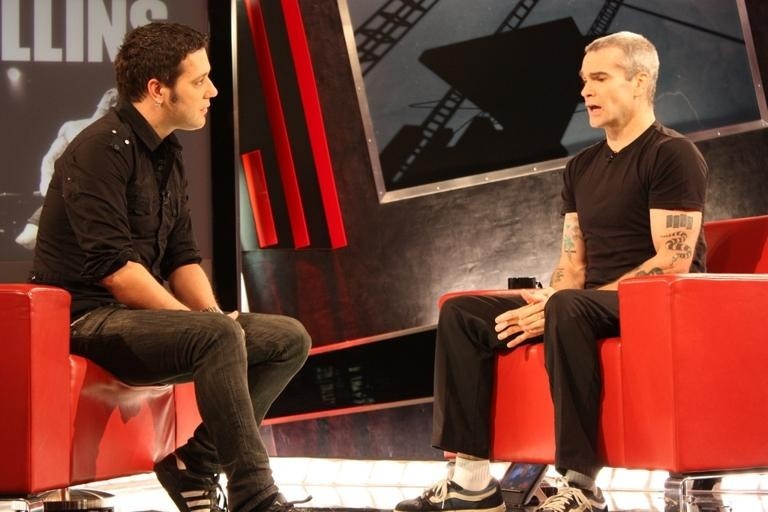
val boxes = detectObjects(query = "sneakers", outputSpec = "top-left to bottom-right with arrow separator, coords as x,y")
393,477 -> 508,511
537,481 -> 609,512
153,452 -> 227,511
251,492 -> 300,512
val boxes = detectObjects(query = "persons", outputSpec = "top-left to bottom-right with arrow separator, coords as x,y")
27,19 -> 319,511
384,25 -> 713,512
11,83 -> 123,252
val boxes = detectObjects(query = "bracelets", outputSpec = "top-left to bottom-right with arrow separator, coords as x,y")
197,305 -> 222,316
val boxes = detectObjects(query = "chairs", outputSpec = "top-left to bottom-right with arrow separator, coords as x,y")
0,280 -> 203,511
434,214 -> 768,512
